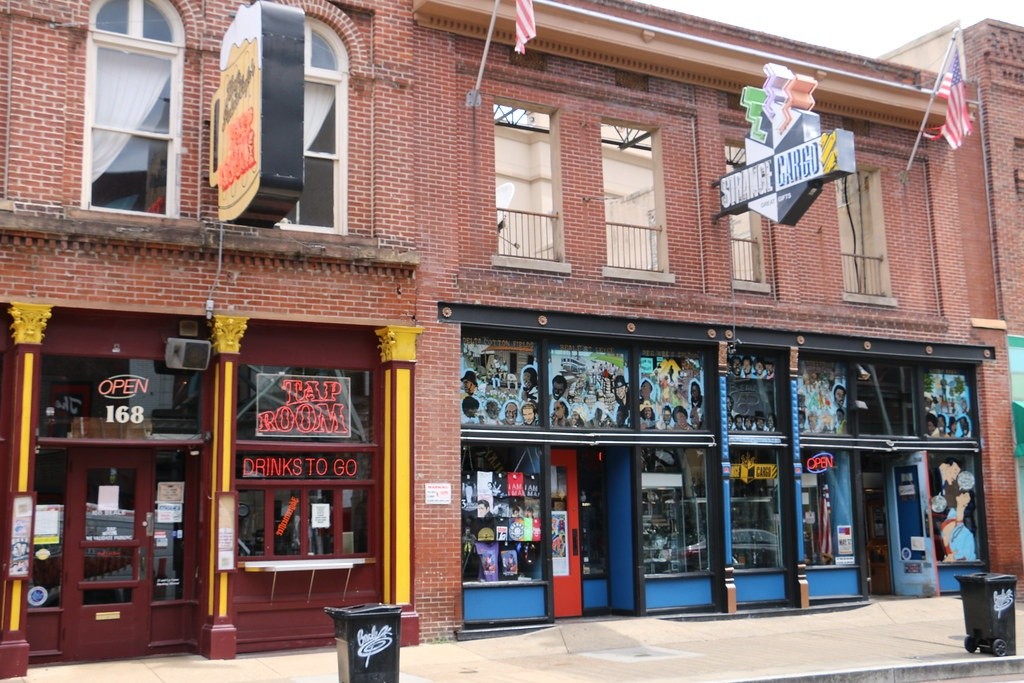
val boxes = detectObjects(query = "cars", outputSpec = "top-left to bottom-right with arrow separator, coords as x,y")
674,529 -> 782,568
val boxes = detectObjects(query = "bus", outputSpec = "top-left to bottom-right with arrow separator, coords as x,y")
676,498 -> 778,546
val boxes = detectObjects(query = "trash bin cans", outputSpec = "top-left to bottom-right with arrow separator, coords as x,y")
323,603 -> 402,683
953,573 -> 1017,657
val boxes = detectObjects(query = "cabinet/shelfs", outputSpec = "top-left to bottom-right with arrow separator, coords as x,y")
460,453 -> 822,582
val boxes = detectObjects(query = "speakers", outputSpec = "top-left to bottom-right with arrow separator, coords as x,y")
165,338 -> 212,370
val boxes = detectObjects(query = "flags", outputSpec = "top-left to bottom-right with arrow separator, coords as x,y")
513,0 -> 536,55
921,40 -> 971,149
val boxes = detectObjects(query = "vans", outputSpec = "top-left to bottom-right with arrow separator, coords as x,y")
34,503 -> 175,599
